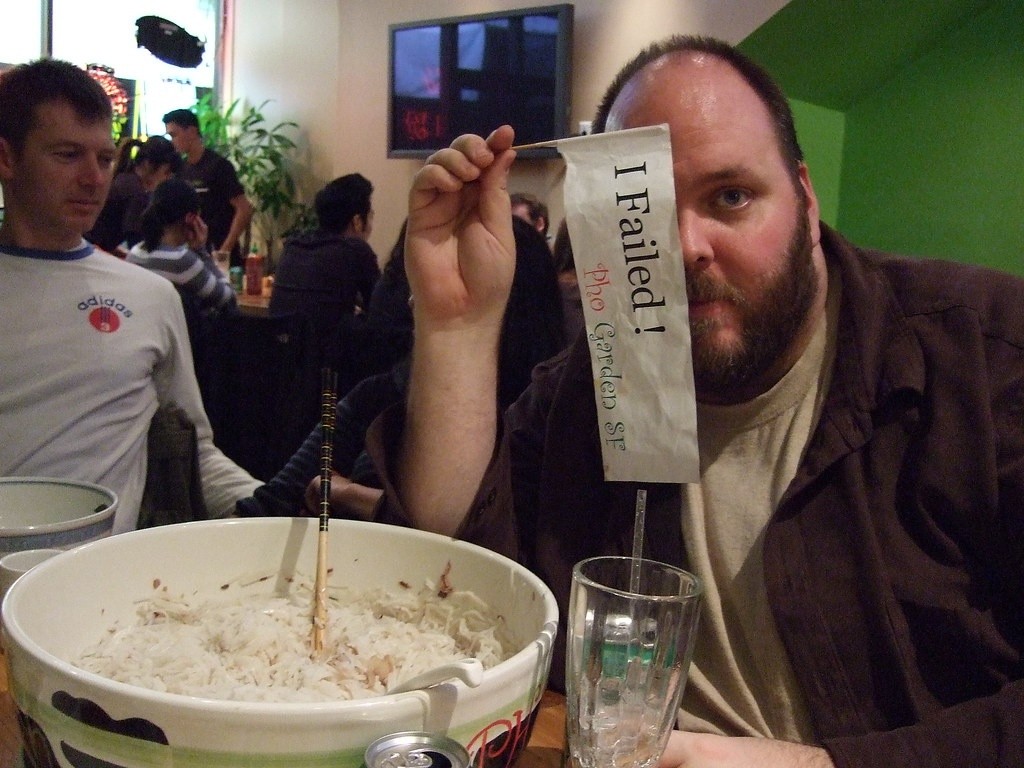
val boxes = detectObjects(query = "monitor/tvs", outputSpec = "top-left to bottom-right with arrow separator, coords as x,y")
386,3 -> 574,159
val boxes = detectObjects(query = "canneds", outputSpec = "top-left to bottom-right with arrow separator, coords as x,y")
229,266 -> 243,295
562,611 -> 663,768
359,730 -> 473,768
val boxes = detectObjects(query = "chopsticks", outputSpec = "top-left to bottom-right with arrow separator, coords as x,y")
311,365 -> 339,653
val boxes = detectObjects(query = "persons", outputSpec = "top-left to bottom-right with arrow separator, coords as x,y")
229,217 -> 567,526
83,135 -> 182,260
124,180 -> 238,317
267,173 -> 382,381
509,193 -> 550,239
365,33 -> 1024,768
162,109 -> 252,268
0,58 -> 260,534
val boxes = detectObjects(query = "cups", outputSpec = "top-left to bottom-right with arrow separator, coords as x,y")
211,250 -> 230,274
561,556 -> 705,768
0,548 -> 65,605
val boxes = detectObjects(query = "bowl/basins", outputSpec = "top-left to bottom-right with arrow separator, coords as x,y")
0,476 -> 118,560
1,516 -> 559,768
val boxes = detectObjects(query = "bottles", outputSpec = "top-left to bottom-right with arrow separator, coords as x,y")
245,241 -> 265,297
